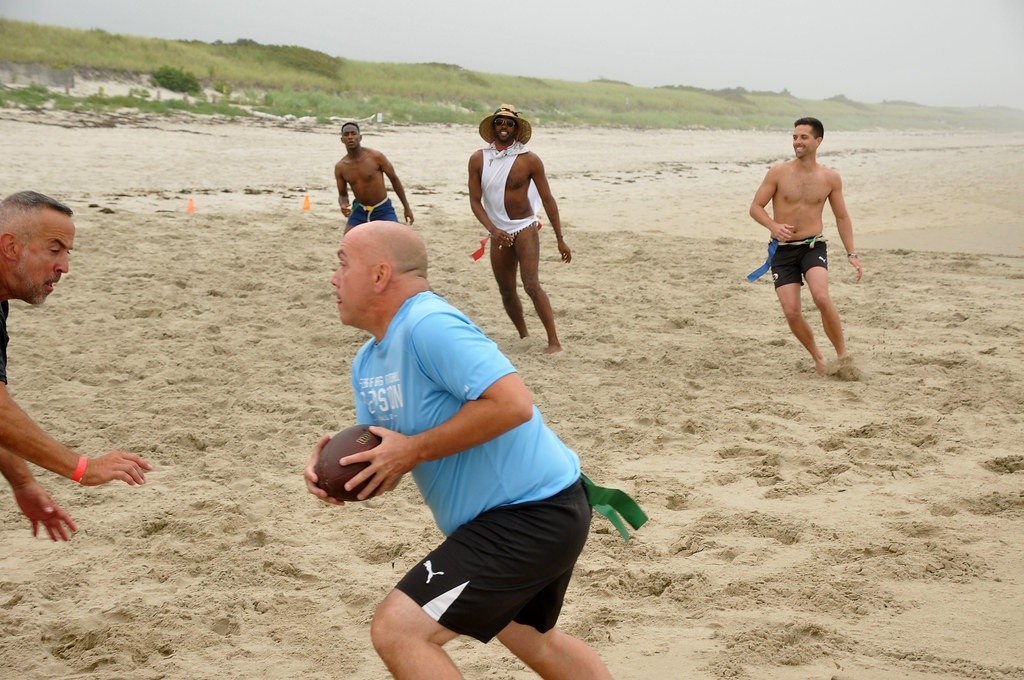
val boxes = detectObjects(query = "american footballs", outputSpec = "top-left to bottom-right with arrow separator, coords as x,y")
314,423 -> 383,505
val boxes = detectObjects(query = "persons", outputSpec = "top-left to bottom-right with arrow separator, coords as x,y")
305,218 -> 618,680
0,190 -> 153,541
467,104 -> 572,353
334,122 -> 414,234
749,117 -> 863,375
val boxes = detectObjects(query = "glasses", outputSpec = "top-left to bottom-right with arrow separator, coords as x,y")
494,118 -> 517,127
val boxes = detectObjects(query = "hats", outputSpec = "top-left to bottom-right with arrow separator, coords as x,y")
478,103 -> 532,145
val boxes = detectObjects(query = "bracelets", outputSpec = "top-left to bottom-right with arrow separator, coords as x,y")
72,457 -> 88,483
556,236 -> 564,239
847,253 -> 859,259
